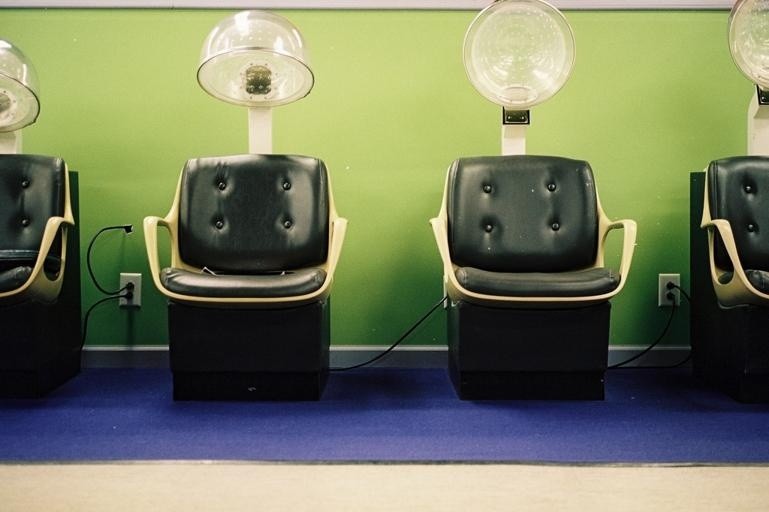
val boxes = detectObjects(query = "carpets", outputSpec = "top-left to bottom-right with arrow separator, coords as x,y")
0,367 -> 769,468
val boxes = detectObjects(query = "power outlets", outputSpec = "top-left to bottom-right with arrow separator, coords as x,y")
658,273 -> 681,307
118,272 -> 143,307
442,276 -> 448,309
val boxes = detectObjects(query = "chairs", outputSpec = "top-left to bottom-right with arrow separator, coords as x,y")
427,153 -> 637,402
142,153 -> 348,400
0,153 -> 83,399
699,154 -> 769,403
